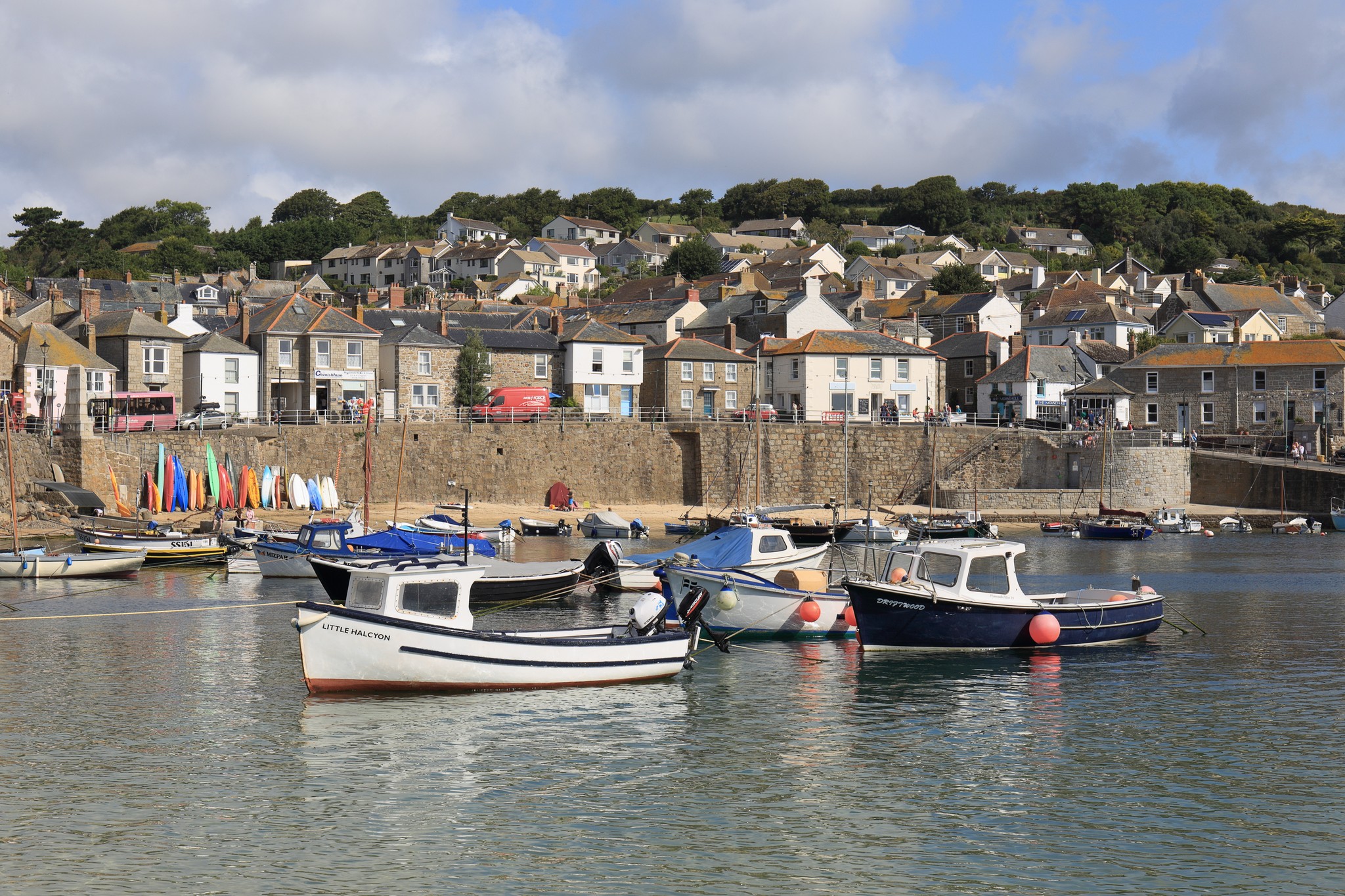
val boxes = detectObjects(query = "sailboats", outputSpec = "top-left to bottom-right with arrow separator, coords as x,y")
0,385 -> 148,579
1070,398 -> 1154,540
1272,470 -> 1291,534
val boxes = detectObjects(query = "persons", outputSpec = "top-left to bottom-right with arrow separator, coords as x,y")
1162,429 -> 1175,446
246,506 -> 258,522
1128,420 -> 1133,430
341,399 -> 347,424
271,411 -> 282,426
685,511 -> 690,526
1088,411 -> 1104,430
792,401 -> 804,425
147,403 -> 165,411
1078,431 -> 1098,447
234,507 -> 244,528
879,402 -> 899,427
213,507 -> 224,530
1290,439 -> 1304,467
8,405 -> 24,432
1188,429 -> 1198,453
1115,418 -> 1121,431
318,392 -> 327,415
923,404 -> 963,427
913,408 -> 921,422
558,488 -> 573,511
91,509 -> 103,517
1239,516 -> 1244,528
1011,409 -> 1017,428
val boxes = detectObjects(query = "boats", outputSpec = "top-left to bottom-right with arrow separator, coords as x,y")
1330,497 -> 1345,531
293,564 -> 702,694
1219,512 -> 1252,534
69,524 -> 229,568
228,398 -> 998,608
1284,524 -> 1302,535
1152,507 -> 1201,533
1289,517 -> 1323,533
1040,489 -> 1078,537
659,553 -> 857,644
834,536 -> 1168,656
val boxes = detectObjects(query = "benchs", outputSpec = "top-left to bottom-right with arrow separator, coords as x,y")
1225,436 -> 1256,448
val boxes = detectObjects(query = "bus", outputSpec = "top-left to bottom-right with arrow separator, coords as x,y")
86,391 -> 176,433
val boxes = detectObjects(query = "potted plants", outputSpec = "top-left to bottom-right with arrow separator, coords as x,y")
1237,429 -> 1249,435
1014,332 -> 1022,335
1273,417 -> 1284,436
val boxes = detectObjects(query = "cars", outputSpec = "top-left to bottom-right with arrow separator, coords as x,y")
170,402 -> 233,431
1333,445 -> 1345,465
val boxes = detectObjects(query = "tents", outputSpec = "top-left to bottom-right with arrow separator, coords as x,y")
548,393 -> 562,407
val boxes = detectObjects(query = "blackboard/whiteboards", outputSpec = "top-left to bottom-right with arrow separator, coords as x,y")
884,398 -> 895,412
858,398 -> 869,415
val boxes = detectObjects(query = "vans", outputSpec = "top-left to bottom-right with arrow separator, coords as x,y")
468,387 -> 550,423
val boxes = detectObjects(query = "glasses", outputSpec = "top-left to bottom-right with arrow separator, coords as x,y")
247,509 -> 249,510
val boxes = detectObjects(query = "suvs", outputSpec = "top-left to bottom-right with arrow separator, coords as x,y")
730,403 -> 778,423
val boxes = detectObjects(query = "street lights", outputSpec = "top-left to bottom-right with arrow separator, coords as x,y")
1128,397 -> 1134,420
1071,348 -> 1080,431
39,339 -> 51,435
